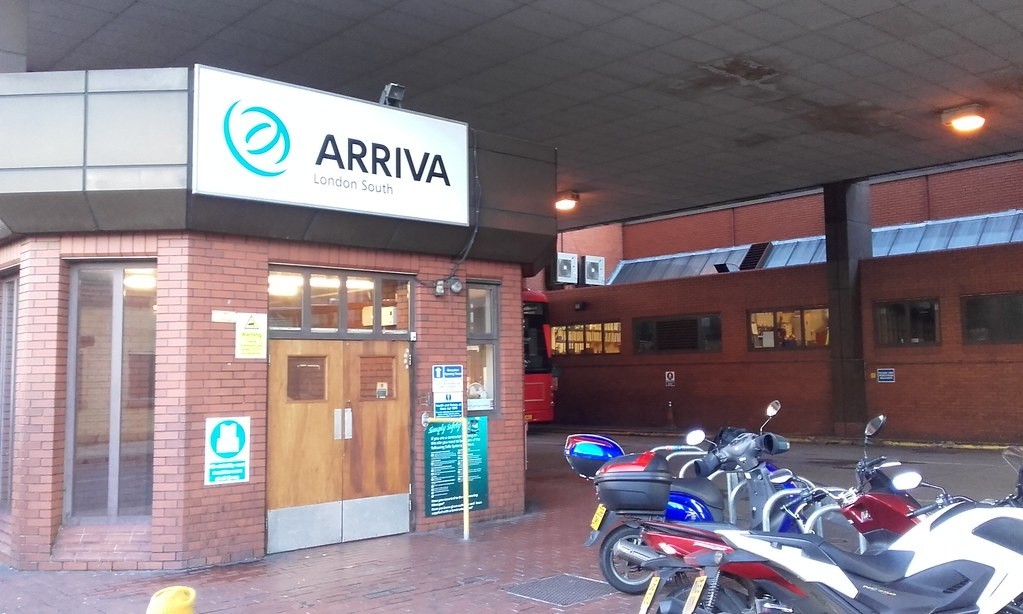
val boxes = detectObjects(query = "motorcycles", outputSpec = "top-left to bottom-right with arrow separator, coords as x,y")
636,446 -> 1023,614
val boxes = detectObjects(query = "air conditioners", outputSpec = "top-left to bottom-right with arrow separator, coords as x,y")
545,249 -> 579,284
575,256 -> 606,288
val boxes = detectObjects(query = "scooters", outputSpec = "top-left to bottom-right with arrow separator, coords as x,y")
564,398 -> 931,596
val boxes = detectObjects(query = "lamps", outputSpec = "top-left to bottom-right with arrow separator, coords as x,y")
381,82 -> 405,108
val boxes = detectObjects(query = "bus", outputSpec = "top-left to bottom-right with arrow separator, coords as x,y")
522,286 -> 558,433
